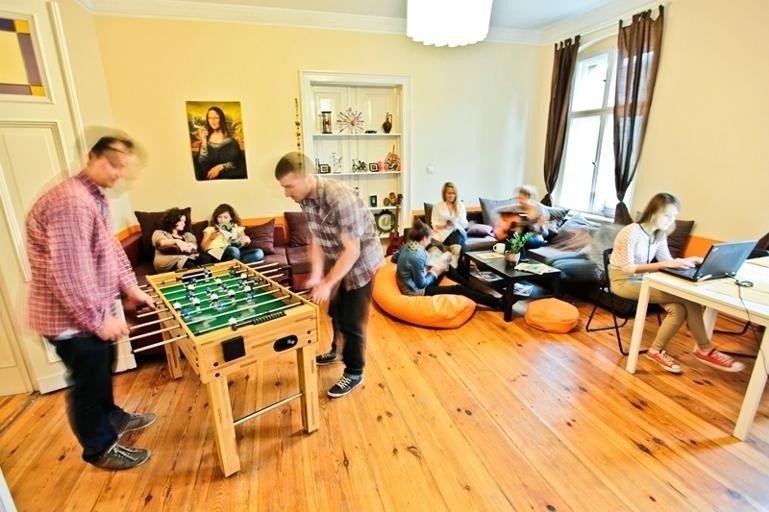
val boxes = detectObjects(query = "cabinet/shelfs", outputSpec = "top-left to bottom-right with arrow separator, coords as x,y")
294,67 -> 412,244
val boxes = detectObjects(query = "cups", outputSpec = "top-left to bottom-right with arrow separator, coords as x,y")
491,242 -> 506,254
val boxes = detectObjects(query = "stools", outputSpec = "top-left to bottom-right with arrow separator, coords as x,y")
523,297 -> 581,334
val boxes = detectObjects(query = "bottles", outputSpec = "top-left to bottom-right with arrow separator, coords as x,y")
383,113 -> 392,133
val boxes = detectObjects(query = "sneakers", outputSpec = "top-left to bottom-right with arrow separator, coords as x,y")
316,352 -> 345,366
694,347 -> 746,372
328,376 -> 364,398
117,412 -> 158,440
92,445 -> 150,470
645,349 -> 682,374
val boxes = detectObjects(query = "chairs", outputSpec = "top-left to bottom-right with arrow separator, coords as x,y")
586,245 -> 670,360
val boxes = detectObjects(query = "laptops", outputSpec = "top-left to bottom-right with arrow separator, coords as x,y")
658,240 -> 757,281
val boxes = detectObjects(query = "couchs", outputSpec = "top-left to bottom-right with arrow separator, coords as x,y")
111,217 -> 317,361
409,202 -> 621,304
371,252 -> 478,330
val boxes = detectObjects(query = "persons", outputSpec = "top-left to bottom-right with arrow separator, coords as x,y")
607,193 -> 746,372
391,182 -> 624,312
276,153 -> 387,398
199,205 -> 265,263
152,209 -> 212,273
198,107 -> 244,180
26,136 -> 157,470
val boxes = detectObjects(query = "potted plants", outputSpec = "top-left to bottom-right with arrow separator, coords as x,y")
503,230 -> 534,267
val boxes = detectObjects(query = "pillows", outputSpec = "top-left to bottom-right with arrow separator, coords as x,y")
552,212 -> 597,247
476,195 -> 519,226
634,212 -> 694,259
284,209 -> 313,249
133,207 -> 195,265
546,205 -> 571,233
423,201 -> 435,226
466,222 -> 493,238
245,216 -> 278,257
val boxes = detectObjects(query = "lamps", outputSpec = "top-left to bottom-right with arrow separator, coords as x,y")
401,1 -> 496,47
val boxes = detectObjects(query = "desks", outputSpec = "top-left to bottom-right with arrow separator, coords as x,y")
620,258 -> 768,443
